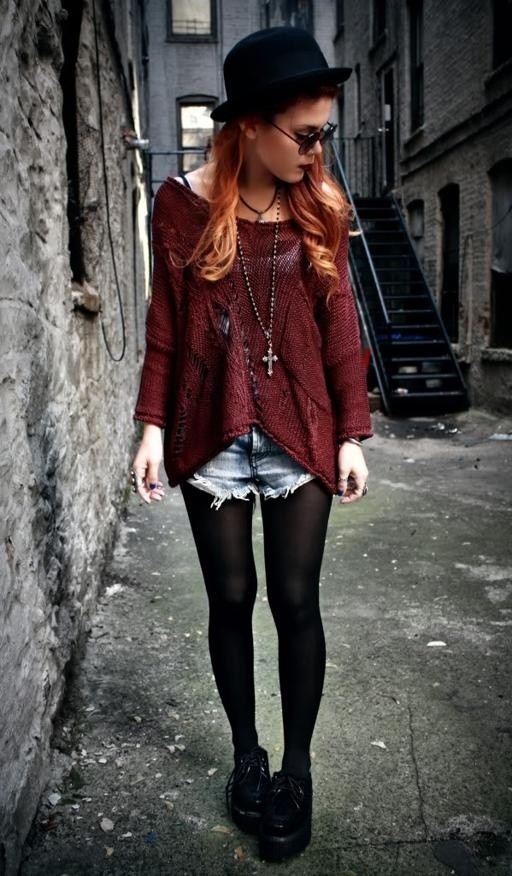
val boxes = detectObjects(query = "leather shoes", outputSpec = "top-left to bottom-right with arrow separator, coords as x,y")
225,746 -> 272,837
257,769 -> 312,864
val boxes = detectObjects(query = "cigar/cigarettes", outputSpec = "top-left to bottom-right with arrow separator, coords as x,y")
139,489 -> 151,507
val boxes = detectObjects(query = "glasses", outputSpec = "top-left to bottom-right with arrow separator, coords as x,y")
263,117 -> 339,156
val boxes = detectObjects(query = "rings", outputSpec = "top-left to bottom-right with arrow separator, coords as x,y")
339,478 -> 348,481
361,484 -> 369,495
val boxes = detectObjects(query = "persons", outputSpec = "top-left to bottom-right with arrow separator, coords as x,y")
132,28 -> 374,862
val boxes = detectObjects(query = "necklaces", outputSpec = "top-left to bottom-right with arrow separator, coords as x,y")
236,188 -> 281,378
235,182 -> 277,223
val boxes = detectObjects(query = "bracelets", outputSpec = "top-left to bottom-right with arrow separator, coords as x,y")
348,437 -> 364,446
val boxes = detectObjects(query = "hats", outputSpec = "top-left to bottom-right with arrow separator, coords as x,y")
210,24 -> 353,123
